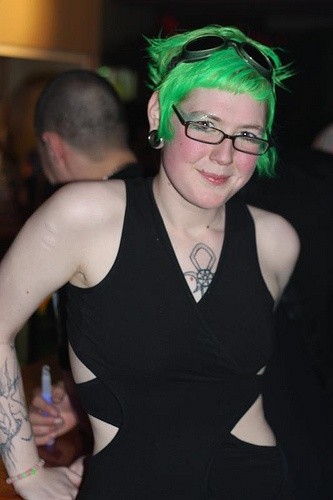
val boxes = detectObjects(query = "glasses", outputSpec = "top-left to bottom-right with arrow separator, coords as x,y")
167,35 -> 276,85
171,106 -> 273,159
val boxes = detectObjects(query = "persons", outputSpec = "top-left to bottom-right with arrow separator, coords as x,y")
35,68 -> 146,372
252,60 -> 333,500
0,27 -> 299,500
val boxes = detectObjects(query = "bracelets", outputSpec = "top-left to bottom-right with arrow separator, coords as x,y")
6,459 -> 45,483
